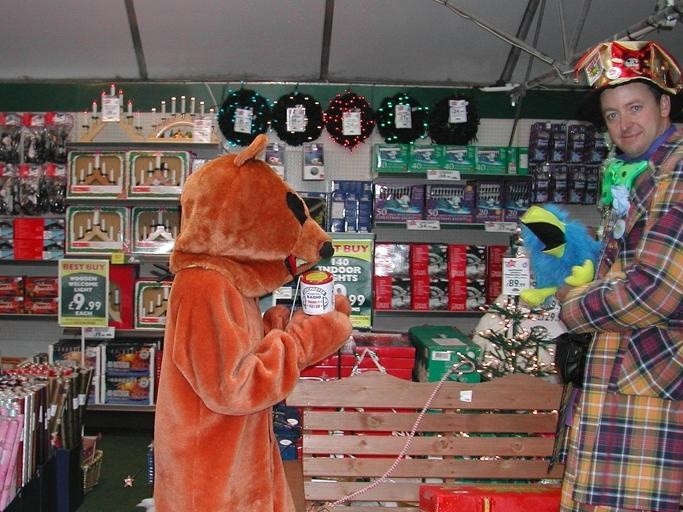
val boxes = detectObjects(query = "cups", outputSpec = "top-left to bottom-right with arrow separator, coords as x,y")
298,270 -> 347,316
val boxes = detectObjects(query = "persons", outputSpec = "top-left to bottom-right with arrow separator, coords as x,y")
546,42 -> 683,511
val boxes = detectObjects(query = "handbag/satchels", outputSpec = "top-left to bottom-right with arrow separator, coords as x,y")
554,332 -> 592,389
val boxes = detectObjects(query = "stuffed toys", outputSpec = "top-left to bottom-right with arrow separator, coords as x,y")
516,200 -> 605,307
152,137 -> 358,511
